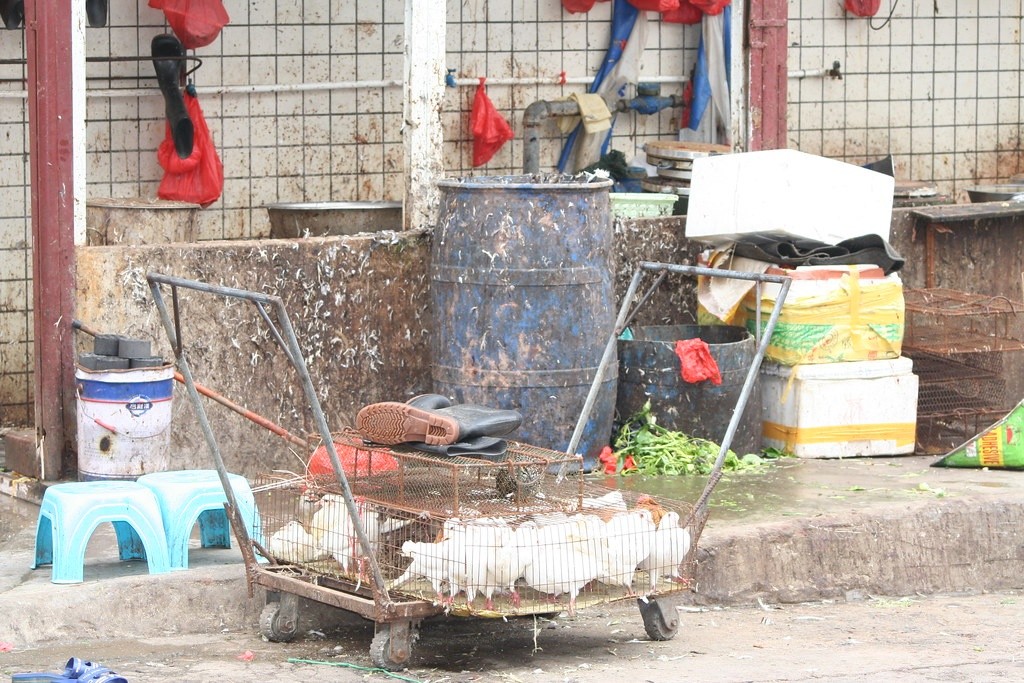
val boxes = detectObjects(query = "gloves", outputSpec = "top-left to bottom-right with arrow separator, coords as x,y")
557,93 -> 612,134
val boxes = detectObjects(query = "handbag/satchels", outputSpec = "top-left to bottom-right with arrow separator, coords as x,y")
310,436 -> 399,485
148,0 -> 230,49
158,90 -> 223,208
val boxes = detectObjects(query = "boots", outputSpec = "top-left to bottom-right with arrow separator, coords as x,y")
356,402 -> 522,444
363,391 -> 508,457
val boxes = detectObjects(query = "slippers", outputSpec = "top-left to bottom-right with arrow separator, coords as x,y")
12,657 -> 129,683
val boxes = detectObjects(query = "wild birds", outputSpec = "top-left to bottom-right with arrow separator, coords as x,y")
263,488 -> 433,593
378,487 -> 692,610
494,465 -> 536,505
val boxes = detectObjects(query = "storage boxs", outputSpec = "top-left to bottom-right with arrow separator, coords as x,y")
759,355 -> 919,459
685,149 -> 895,249
698,250 -> 905,364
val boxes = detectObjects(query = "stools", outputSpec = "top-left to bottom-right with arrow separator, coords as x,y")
137,470 -> 267,570
30,481 -> 172,584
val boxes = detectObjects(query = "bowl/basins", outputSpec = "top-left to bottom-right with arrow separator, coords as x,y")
262,199 -> 404,237
609,190 -> 679,220
963,182 -> 1024,204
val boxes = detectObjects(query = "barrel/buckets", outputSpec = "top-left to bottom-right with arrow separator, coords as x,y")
614,326 -> 765,463
66,361 -> 175,485
88,197 -> 200,248
426,173 -> 620,475
